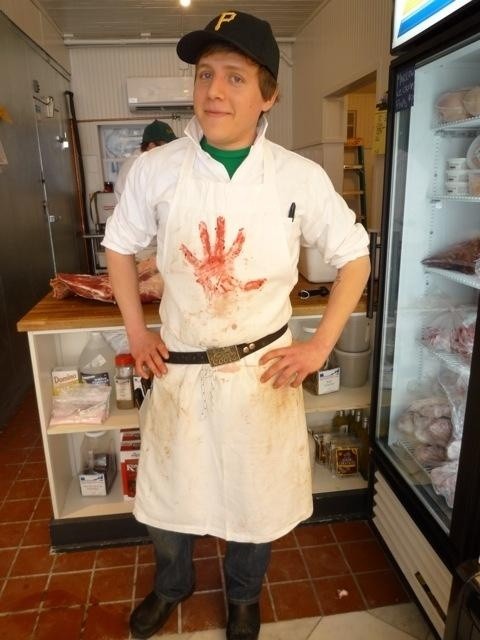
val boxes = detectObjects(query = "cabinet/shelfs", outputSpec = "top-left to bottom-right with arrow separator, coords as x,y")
395,110 -> 479,520
17,287 -> 377,554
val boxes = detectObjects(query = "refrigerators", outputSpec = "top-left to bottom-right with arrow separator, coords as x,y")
368,0 -> 480,640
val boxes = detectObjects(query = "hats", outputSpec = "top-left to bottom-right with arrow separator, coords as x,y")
142,119 -> 179,143
176,10 -> 279,81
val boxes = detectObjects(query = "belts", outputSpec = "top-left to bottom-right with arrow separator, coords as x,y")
163,321 -> 289,368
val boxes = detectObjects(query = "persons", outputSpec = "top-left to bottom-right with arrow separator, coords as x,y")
100,11 -> 371,640
113,119 -> 179,200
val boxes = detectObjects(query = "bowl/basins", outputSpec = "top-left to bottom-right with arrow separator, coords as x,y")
446,181 -> 467,195
446,170 -> 467,181
447,157 -> 467,169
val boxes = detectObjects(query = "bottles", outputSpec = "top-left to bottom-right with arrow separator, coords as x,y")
332,408 -> 371,483
110,353 -> 135,408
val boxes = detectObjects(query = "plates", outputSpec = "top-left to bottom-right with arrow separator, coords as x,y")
467,135 -> 480,170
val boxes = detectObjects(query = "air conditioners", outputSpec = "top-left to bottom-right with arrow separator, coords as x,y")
124,75 -> 195,116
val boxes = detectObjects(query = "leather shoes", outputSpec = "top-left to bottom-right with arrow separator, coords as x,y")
130,582 -> 196,639
225,602 -> 261,640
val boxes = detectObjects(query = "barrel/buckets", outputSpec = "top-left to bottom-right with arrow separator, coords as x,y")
80,331 -> 117,403
296,322 -> 340,395
77,431 -> 118,496
